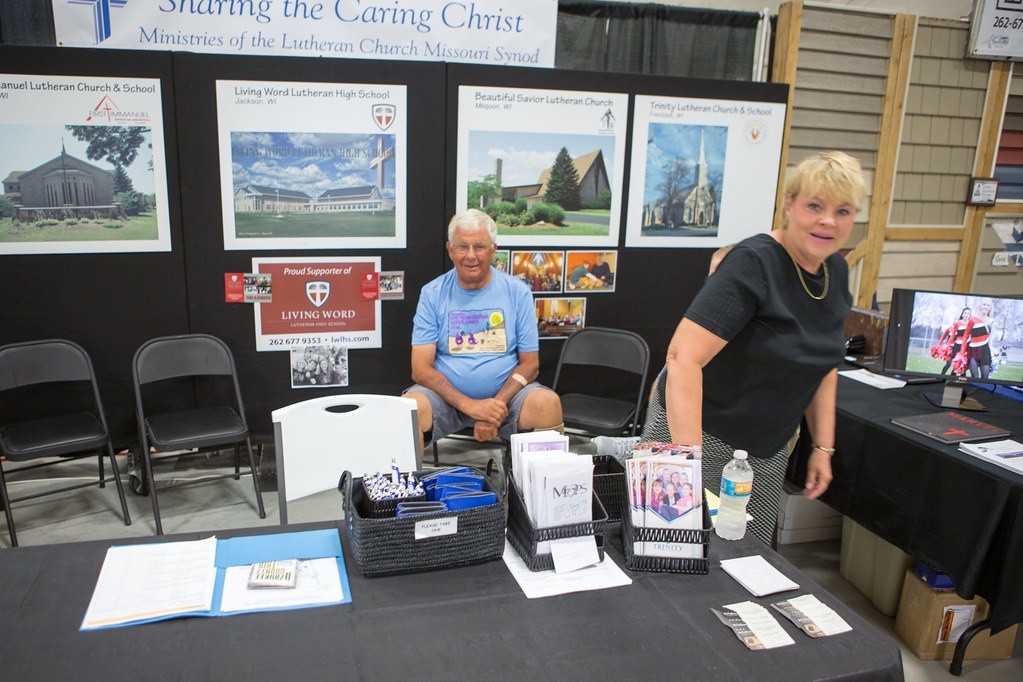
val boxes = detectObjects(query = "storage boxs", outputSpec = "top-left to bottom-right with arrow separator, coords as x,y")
777,477 -> 1018,661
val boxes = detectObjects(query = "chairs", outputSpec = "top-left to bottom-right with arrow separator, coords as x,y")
131,334 -> 265,536
0,337 -> 130,548
271,394 -> 423,526
421,327 -> 650,473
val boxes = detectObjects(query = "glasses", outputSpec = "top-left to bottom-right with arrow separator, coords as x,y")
450,240 -> 495,254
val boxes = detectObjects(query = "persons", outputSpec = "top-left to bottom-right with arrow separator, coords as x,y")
937,299 -> 995,380
401,209 -> 565,463
293,355 -> 347,385
631,462 -> 693,521
590,435 -> 641,466
568,257 -> 610,290
538,314 -> 579,337
492,259 -> 507,274
641,150 -> 869,546
520,272 -> 560,291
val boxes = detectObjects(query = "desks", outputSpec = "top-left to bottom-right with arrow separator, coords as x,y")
0,520 -> 907,682
787,353 -> 1023,677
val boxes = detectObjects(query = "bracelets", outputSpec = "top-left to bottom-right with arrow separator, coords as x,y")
813,444 -> 835,456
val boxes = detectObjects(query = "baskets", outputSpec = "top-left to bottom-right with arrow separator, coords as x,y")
507,455 -> 714,575
338,456 -> 507,578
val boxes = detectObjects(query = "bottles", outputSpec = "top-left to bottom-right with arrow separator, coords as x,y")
715,450 -> 753,541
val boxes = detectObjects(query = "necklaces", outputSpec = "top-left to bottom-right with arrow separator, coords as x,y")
782,229 -> 830,299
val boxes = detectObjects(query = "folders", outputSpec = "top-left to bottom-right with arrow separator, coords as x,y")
80,527 -> 352,633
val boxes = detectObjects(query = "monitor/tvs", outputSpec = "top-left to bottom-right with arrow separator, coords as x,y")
882,288 -> 1023,411
